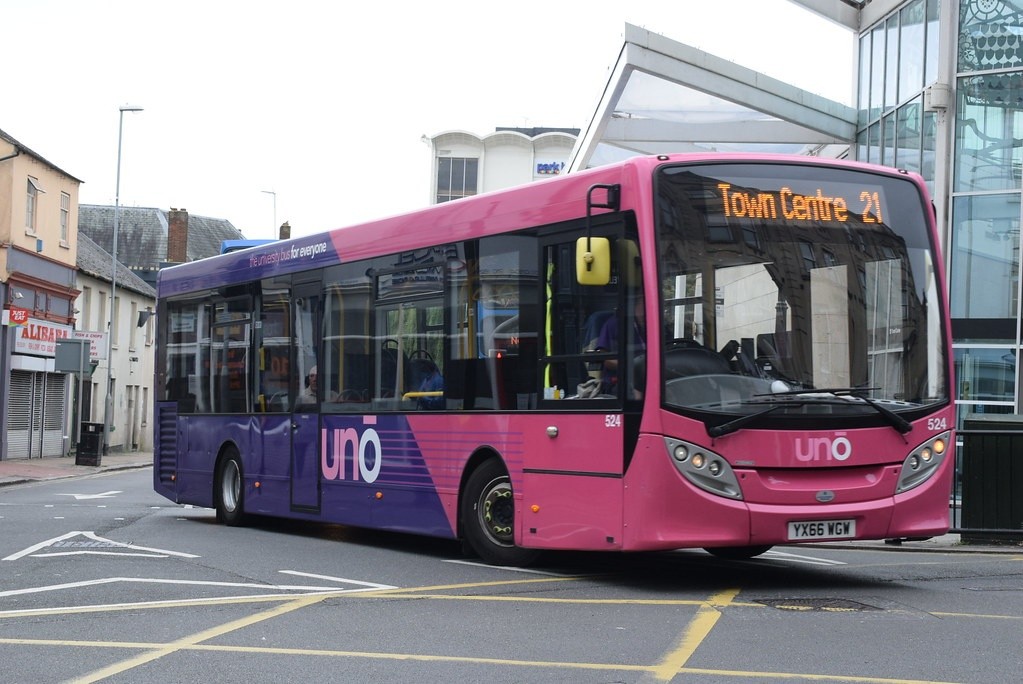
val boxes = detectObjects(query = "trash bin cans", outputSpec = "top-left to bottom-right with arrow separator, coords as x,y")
961,414 -> 1023,544
75,421 -> 105,466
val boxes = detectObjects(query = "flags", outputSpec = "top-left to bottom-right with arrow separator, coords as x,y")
137,312 -> 151,328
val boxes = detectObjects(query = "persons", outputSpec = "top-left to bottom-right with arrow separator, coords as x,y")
295,364 -> 343,404
594,285 -> 665,397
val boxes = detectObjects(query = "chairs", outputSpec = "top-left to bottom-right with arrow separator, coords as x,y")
166,309 -> 617,413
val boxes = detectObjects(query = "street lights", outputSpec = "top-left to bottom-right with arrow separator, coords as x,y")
104,103 -> 143,453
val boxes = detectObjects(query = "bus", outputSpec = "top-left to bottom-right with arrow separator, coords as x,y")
153,151 -> 961,564
